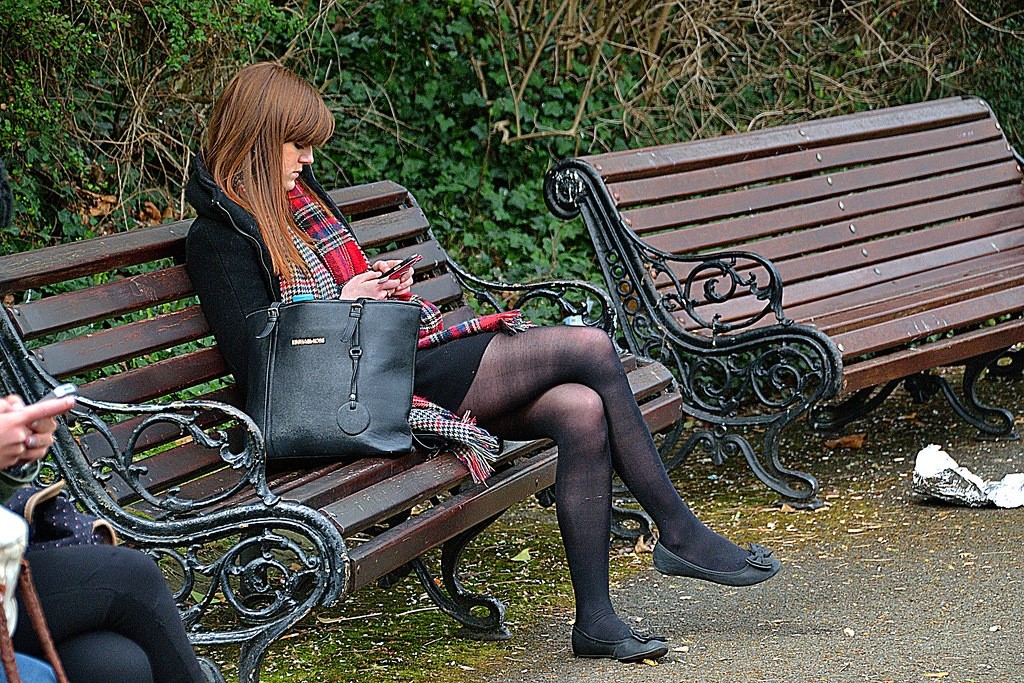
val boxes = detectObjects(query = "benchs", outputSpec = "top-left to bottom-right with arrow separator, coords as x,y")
0,179 -> 687,683
542,94 -> 1024,500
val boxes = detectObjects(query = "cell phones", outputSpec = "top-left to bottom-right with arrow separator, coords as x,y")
376,253 -> 417,278
36,382 -> 80,405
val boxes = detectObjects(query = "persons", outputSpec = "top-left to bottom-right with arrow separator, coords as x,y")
0,395 -> 206,683
185,62 -> 781,660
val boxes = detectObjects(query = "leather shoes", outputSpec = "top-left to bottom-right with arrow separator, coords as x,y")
568,622 -> 668,662
652,537 -> 783,588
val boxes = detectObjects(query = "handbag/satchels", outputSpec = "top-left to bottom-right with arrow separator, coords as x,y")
0,508 -> 31,647
5,478 -> 117,546
246,300 -> 422,461
